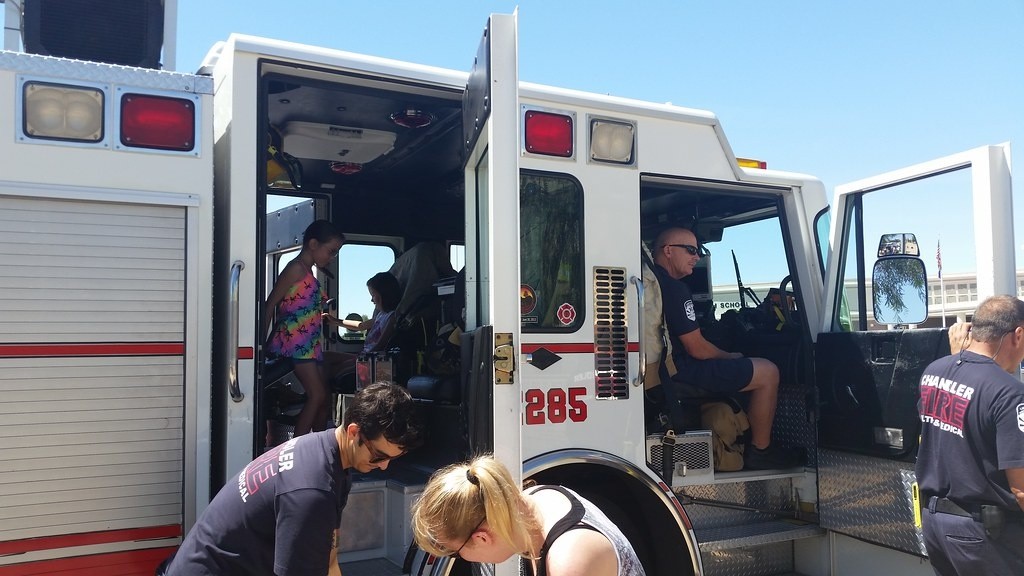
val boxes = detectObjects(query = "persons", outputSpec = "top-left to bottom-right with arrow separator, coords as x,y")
915,295 -> 1024,576
322,271 -> 401,395
651,227 -> 800,471
265,219 -> 344,437
165,380 -> 424,576
411,453 -> 645,576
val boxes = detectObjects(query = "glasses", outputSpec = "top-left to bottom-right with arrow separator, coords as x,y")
448,516 -> 486,559
323,245 -> 339,258
662,245 -> 702,257
358,431 -> 408,464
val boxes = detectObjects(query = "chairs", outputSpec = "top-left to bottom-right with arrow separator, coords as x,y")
334,242 -> 465,405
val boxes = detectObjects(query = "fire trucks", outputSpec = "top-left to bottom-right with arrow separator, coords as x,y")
0,4 -> 1024,576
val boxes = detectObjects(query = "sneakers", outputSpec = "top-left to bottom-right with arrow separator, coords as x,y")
746,442 -> 785,470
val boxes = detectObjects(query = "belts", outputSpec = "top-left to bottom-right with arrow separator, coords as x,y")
924,495 -> 1024,521
